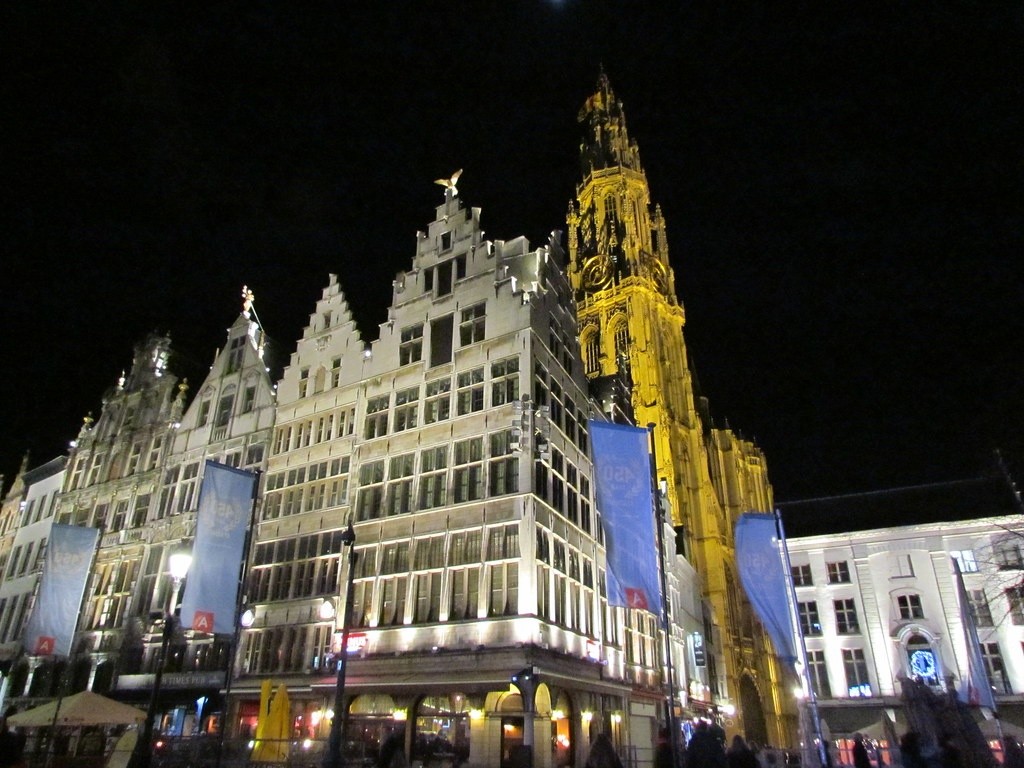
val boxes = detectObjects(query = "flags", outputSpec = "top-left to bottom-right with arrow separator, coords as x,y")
179,459 -> 257,636
22,523 -> 100,657
589,419 -> 667,628
736,513 -> 798,660
951,556 -> 999,711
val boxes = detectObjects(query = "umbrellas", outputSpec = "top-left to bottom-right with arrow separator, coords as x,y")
7,689 -> 148,760
251,680 -> 291,762
882,677 -> 1003,767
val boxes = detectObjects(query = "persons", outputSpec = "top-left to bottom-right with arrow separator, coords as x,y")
653,717 -> 762,768
851,736 -> 870,768
585,733 -> 624,768
